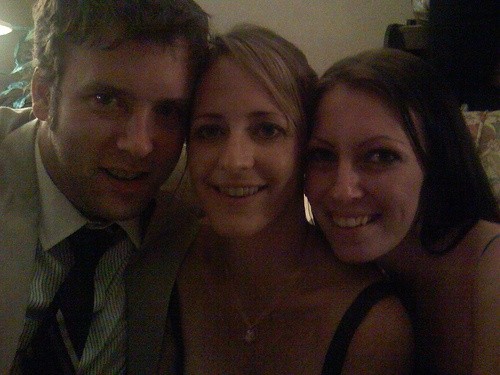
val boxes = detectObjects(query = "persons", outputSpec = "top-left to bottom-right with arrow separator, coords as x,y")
304,48 -> 500,375
157,25 -> 414,375
0,0 -> 208,375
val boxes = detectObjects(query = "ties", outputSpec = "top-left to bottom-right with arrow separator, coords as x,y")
19,224 -> 125,375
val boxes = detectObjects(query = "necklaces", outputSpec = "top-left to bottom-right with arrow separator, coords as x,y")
221,241 -> 309,345
